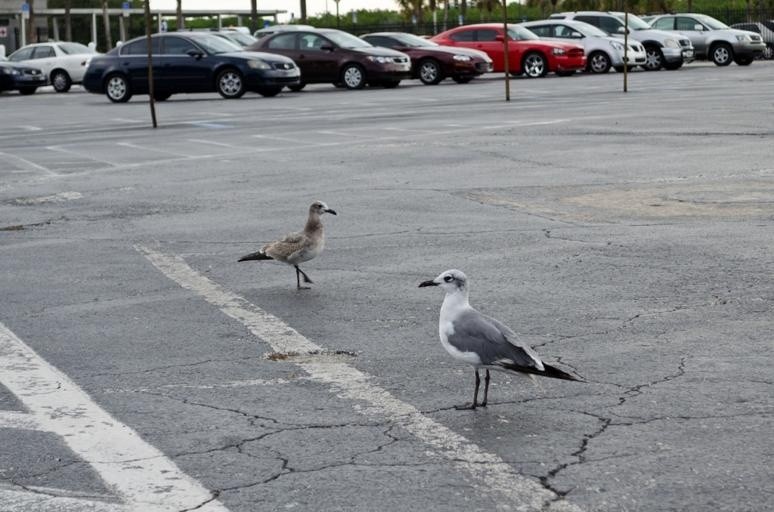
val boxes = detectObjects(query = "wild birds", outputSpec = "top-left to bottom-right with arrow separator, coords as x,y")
237,199 -> 337,290
418,268 -> 590,410
